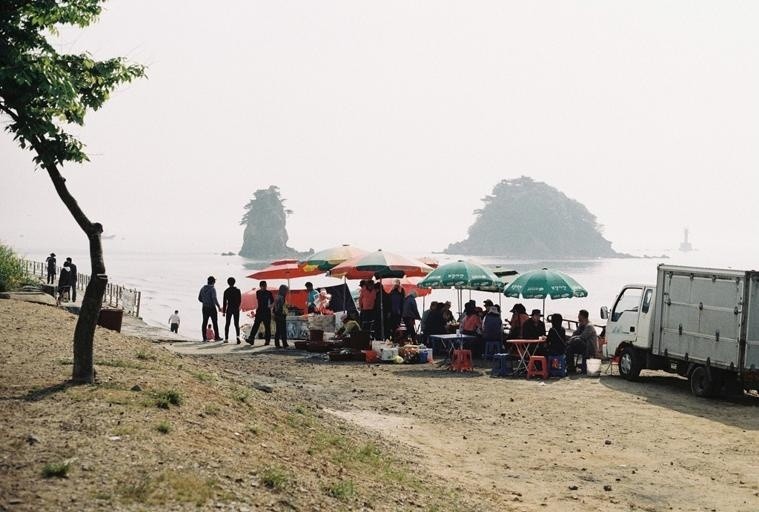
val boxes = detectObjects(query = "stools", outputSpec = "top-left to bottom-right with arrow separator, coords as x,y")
527,356 -> 549,380
490,353 -> 514,378
575,352 -> 595,375
548,354 -> 568,379
485,341 -> 500,359
453,348 -> 473,375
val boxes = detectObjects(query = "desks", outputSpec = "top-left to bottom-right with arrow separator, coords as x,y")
506,339 -> 547,377
430,334 -> 477,371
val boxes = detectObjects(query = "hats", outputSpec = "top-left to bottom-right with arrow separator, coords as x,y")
488,307 -> 499,315
528,310 -> 544,317
339,314 -> 347,322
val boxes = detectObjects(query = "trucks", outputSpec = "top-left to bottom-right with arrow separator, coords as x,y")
598,260 -> 759,406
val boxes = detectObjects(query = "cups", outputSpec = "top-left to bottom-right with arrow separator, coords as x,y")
539,336 -> 542,341
456,328 -> 460,336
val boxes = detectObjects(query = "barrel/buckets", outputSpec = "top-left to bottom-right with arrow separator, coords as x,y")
585,358 -> 601,377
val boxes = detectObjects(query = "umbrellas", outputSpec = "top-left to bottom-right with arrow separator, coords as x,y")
503,266 -> 588,322
297,244 -> 371,313
416,260 -> 503,315
219,287 -> 257,312
325,249 -> 436,337
246,258 -> 325,306
492,265 -> 520,306
419,258 -> 439,312
375,277 -> 431,297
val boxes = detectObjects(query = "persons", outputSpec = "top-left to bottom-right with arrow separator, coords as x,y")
66,257 -> 77,302
168,310 -> 180,333
329,289 -> 348,330
206,324 -> 215,340
198,276 -> 223,341
247,311 -> 256,318
223,277 -> 241,344
58,261 -> 72,298
46,253 -> 56,284
305,281 -> 320,314
358,279 -> 599,373
245,281 -> 274,346
273,284 -> 289,348
308,288 -> 333,315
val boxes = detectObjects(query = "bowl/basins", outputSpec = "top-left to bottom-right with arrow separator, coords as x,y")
327,351 -> 352,360
293,340 -> 333,352
